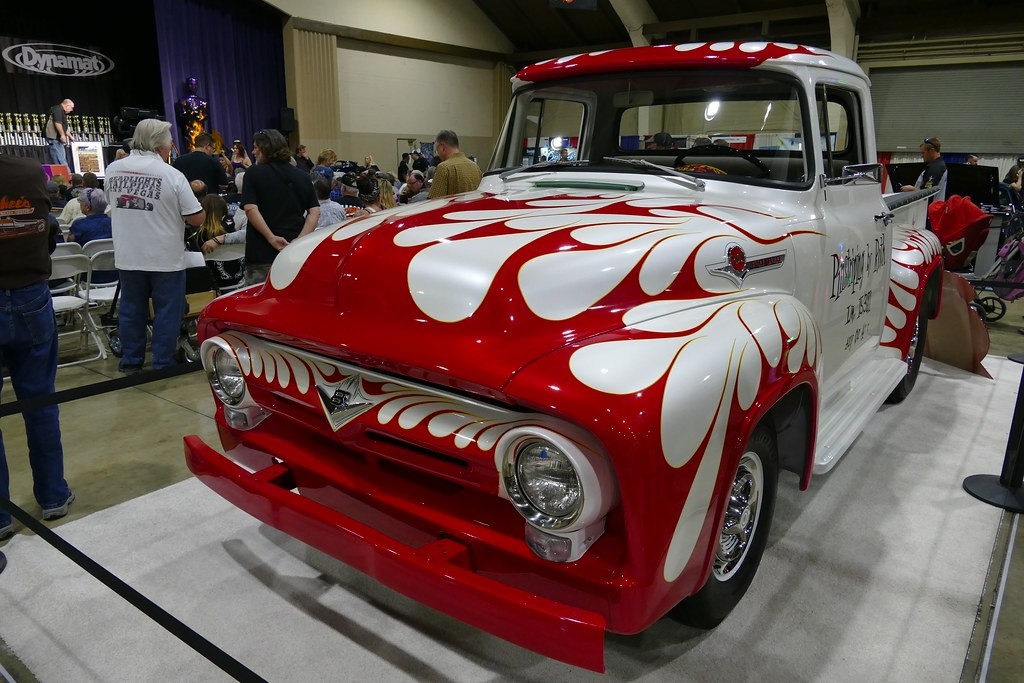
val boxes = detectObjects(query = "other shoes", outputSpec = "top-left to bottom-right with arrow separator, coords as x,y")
118,361 -> 142,373
0,521 -> 15,539
42,491 -> 75,519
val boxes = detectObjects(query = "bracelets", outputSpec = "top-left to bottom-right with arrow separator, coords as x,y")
212,237 -> 223,245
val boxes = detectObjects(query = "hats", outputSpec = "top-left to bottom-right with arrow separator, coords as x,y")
337,173 -> 357,188
48,182 -> 58,193
645,132 -> 672,146
1018,158 -> 1024,164
409,150 -> 418,155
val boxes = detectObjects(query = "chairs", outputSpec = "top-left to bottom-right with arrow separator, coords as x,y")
46,219 -> 217,367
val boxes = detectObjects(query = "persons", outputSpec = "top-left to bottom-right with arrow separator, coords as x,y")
899,138 -> 947,231
240,129 -> 321,289
540,156 -> 547,163
1001,158 -> 1024,239
173,131 -> 401,298
40,99 -> 75,174
0,153 -> 75,542
103,119 -> 206,373
114,149 -> 128,162
646,131 -> 678,149
548,154 -> 552,161
713,139 -> 729,148
176,77 -> 207,153
559,149 -> 569,161
428,130 -> 483,200
44,172 -> 118,327
397,150 -> 442,205
693,138 -> 712,149
967,154 -> 978,165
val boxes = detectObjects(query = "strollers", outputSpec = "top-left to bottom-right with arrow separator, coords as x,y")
100,226 -> 221,371
928,195 -> 1024,322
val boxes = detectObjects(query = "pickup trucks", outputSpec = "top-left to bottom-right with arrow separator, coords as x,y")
184,42 -> 946,674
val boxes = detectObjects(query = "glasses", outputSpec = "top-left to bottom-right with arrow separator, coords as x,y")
434,145 -> 444,154
76,199 -> 84,202
206,144 -> 216,153
259,128 -> 267,136
924,138 -> 938,149
409,179 -> 418,184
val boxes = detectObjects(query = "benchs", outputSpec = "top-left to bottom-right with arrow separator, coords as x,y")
613,154 -> 848,184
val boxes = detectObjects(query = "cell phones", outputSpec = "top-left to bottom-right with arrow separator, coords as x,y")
898,183 -> 903,187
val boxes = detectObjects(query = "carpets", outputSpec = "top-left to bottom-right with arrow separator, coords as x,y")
0,354 -> 1023,683
